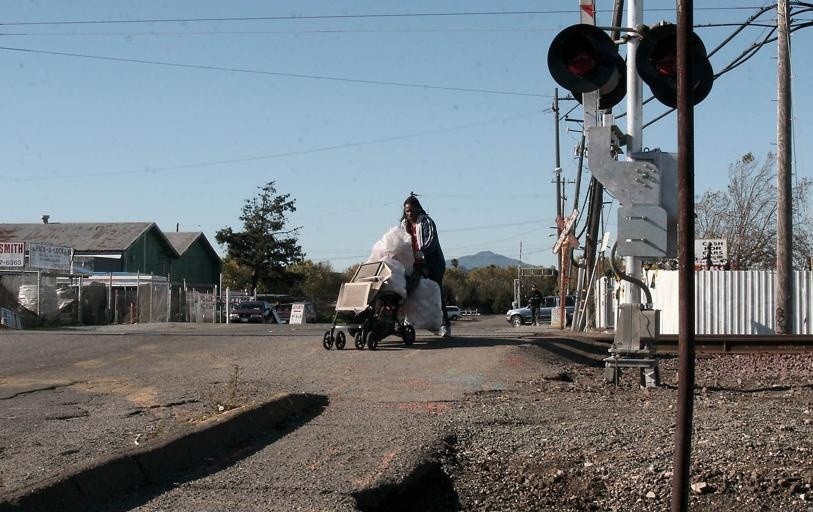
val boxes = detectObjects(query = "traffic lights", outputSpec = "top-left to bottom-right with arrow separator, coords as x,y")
549,24 -> 616,89
636,24 -> 708,91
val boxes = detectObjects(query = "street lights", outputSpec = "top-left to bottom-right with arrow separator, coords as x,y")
552,167 -> 565,288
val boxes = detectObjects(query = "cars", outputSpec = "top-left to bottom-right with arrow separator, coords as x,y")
186,292 -> 336,325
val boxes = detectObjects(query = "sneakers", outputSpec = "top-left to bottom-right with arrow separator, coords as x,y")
531,321 -> 540,326
427,324 -> 452,338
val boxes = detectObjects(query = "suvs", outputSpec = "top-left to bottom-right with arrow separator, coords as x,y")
504,294 -> 581,328
445,303 -> 462,322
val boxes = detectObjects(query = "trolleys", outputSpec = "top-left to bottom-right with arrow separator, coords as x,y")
322,252 -> 422,350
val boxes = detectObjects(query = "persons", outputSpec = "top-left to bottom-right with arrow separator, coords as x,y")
398,191 -> 452,349
527,284 -> 546,328
